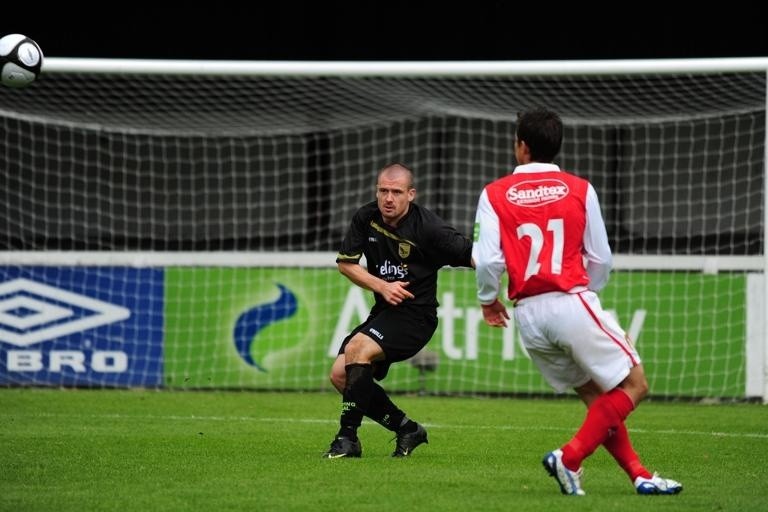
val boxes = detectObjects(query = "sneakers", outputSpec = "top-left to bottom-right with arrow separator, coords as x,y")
542,448 -> 586,496
633,470 -> 684,495
392,423 -> 429,457
328,437 -> 362,459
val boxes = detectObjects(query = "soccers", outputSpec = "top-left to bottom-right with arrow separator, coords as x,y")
0,34 -> 43,87
